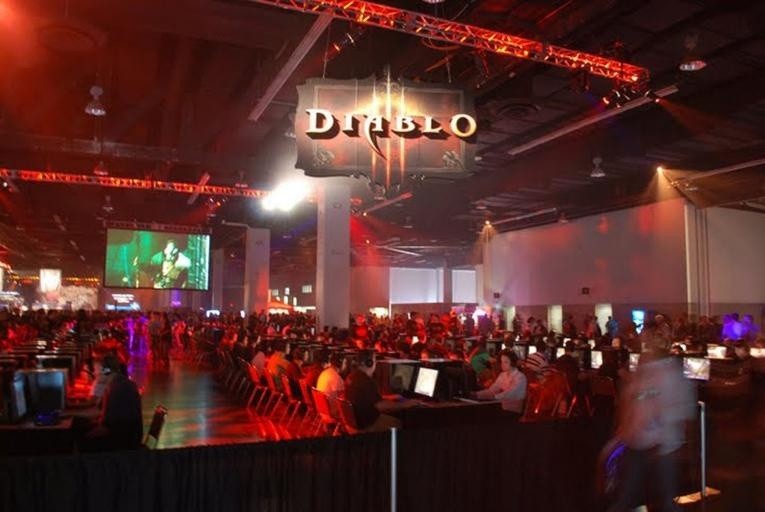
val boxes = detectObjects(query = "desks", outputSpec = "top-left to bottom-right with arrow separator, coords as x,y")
0,415 -> 73,430
374,398 -> 501,428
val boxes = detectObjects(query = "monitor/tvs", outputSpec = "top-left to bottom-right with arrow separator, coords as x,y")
562,338 -> 571,347
554,347 -> 566,359
627,351 -> 641,373
589,350 -> 604,369
706,346 -> 728,360
749,346 -> 765,359
681,356 -> 713,382
499,334 -> 549,356
411,335 -> 420,344
408,362 -> 444,401
587,340 -> 596,350
0,371 -> 32,421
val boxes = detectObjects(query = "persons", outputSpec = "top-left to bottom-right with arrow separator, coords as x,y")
0,300 -> 763,512
134,240 -> 191,289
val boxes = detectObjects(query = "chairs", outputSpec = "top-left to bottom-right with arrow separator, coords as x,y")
518,367 -> 615,422
141,405 -> 168,449
191,336 -> 367,438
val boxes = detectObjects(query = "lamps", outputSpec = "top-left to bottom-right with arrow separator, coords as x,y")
216,197 -> 230,206
332,25 -> 369,54
668,35 -> 707,73
84,86 -> 111,116
94,160 -> 109,176
602,82 -> 660,108
102,196 -> 114,211
559,212 -> 569,223
590,158 -> 606,178
